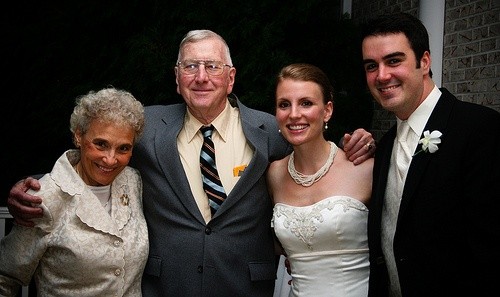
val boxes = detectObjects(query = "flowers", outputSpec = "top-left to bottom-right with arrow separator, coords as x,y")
412,130 -> 442,157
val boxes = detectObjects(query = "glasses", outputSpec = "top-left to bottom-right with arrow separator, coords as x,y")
176,60 -> 233,76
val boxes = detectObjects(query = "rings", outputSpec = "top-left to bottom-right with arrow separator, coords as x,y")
366,143 -> 373,150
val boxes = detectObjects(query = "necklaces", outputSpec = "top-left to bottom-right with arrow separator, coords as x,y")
74,162 -> 111,207
287,140 -> 338,187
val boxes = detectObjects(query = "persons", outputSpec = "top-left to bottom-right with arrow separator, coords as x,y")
283,13 -> 500,297
0,85 -> 151,297
266,64 -> 375,297
8,29 -> 375,297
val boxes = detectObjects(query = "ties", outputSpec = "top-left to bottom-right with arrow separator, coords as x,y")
199,124 -> 228,219
395,123 -> 412,182
